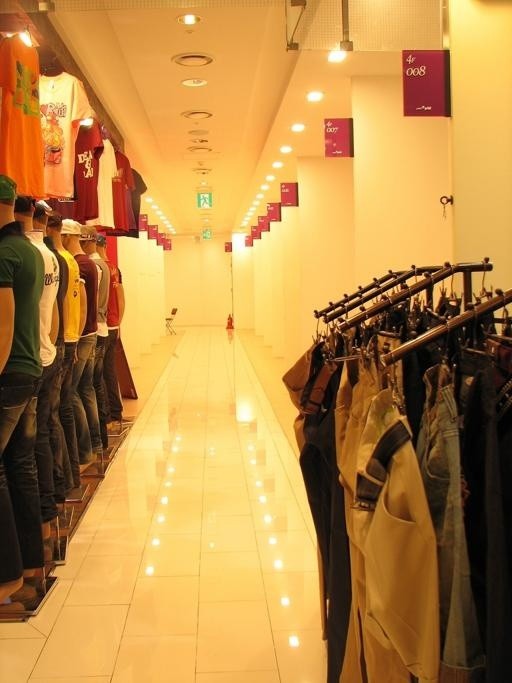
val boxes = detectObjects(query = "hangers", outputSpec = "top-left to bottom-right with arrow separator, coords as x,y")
19,18 -> 33,45
40,55 -> 62,76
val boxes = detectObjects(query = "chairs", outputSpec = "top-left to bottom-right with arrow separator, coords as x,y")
166,307 -> 179,336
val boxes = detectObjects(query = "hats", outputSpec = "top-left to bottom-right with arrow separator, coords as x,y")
95,234 -> 108,247
79,225 -> 97,241
0,173 -> 18,202
13,194 -> 38,213
45,209 -> 63,228
33,202 -> 51,220
61,218 -> 82,235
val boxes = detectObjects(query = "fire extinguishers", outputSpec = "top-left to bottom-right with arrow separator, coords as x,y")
226,314 -> 234,329
